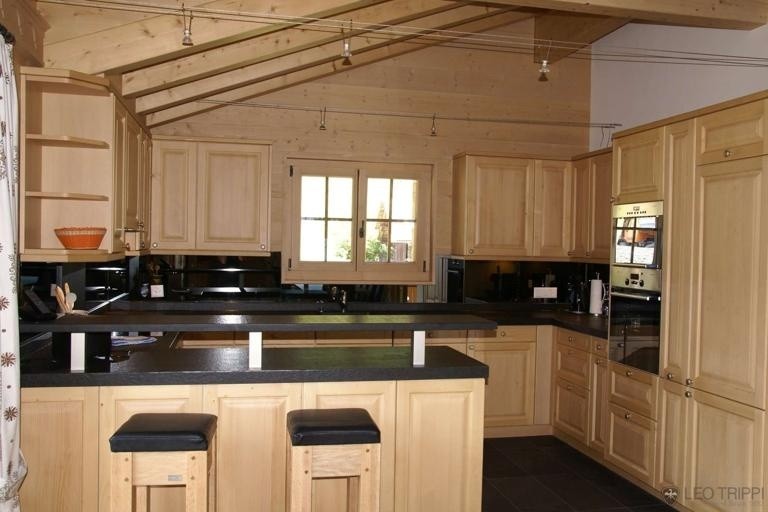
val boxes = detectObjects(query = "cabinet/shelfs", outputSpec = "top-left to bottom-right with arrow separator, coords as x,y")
393,89 -> 768,512
151,135 -> 197,255
18,68 -> 151,264
197,136 -> 273,257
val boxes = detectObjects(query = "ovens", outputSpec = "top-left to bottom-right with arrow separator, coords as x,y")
610,202 -> 663,377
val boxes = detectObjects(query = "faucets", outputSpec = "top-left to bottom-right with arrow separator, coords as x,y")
331,288 -> 348,313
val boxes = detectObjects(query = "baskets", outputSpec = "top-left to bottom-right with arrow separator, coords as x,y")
54,227 -> 107,250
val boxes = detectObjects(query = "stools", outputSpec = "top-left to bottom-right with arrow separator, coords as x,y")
287,408 -> 380,512
110,413 -> 217,512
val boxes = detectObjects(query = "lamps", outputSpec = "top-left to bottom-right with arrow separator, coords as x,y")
191,98 -> 623,136
52,1 -> 768,73
